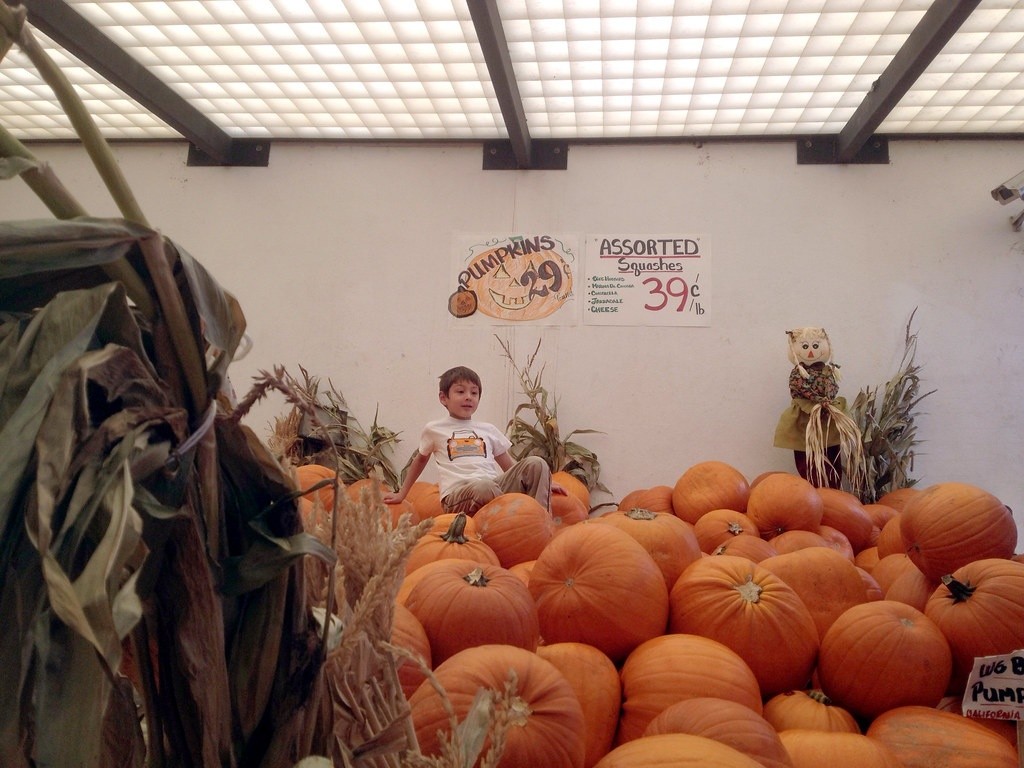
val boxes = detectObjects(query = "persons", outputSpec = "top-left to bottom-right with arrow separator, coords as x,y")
783,324 -> 843,491
379,365 -> 567,521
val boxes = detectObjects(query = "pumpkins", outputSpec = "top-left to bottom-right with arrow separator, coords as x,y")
289,462 -> 1024,767
